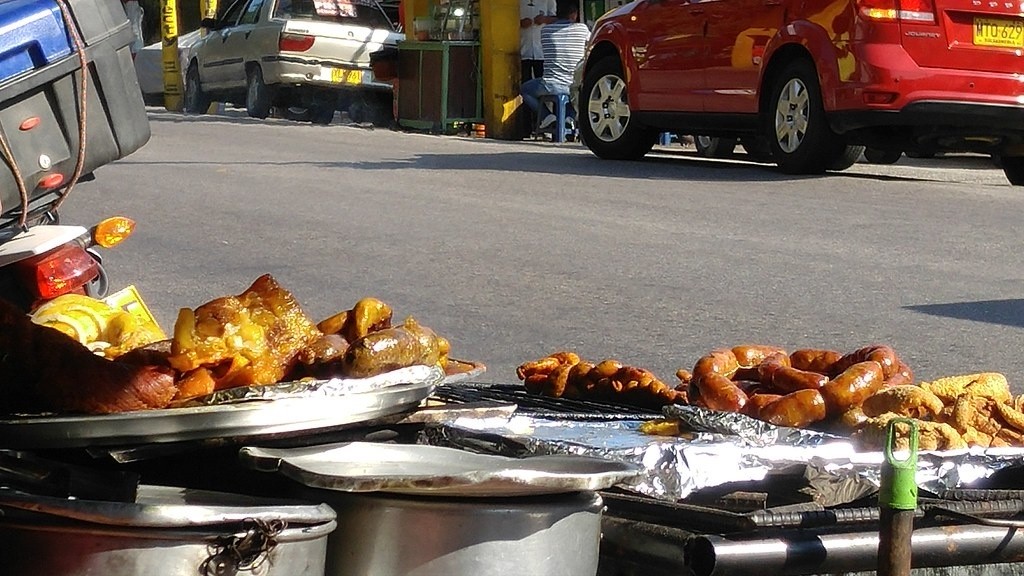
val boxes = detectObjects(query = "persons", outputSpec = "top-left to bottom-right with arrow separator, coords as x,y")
519,0 -> 592,134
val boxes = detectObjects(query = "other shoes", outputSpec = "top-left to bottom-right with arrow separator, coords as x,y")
539,114 -> 557,129
565,116 -> 575,141
522,132 -> 543,140
544,132 -> 552,139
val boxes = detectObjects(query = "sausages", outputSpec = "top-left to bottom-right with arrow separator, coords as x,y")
516,343 -> 915,430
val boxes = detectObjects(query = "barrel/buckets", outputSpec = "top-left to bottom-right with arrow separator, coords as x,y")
0,515 -> 338,576
302,484 -> 608,576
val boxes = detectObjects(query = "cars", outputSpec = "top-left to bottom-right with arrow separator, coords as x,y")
185,0 -> 408,123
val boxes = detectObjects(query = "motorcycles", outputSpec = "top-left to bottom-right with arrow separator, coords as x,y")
0,0 -> 166,352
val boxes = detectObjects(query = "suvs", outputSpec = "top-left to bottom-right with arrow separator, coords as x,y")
577,0 -> 1024,187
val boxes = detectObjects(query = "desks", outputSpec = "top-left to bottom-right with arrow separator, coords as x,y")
521,58 -> 544,79
397,40 -> 483,131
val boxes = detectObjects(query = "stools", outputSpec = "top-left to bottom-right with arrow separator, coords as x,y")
535,95 -> 579,142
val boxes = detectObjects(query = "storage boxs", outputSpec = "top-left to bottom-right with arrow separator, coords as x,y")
0,0 -> 150,216
